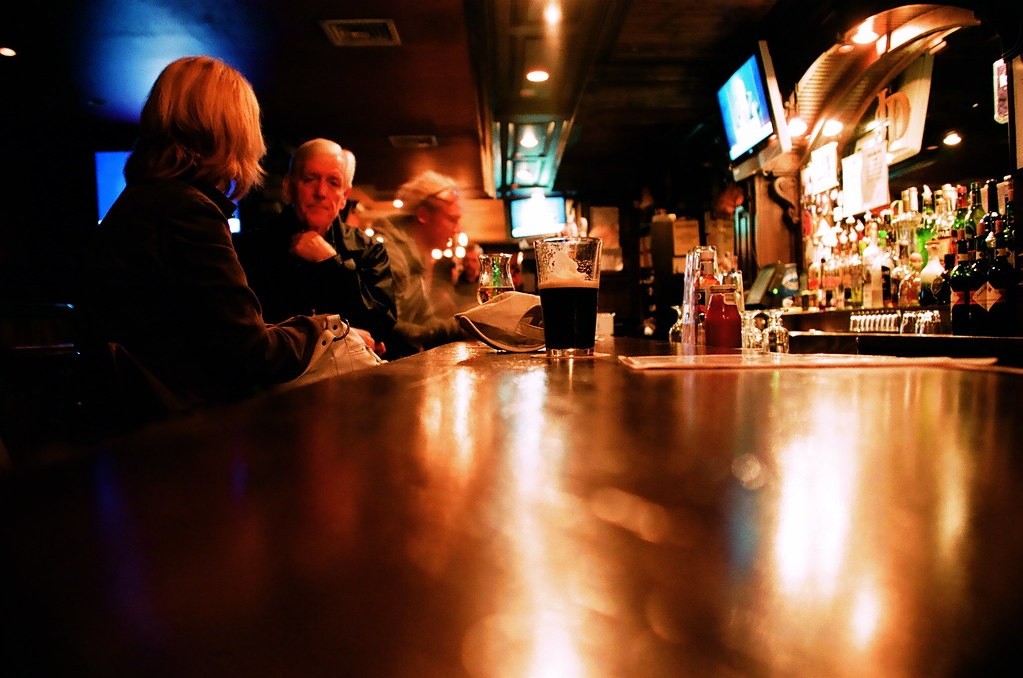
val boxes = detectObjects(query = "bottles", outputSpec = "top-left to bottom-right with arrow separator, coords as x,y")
781,174 -> 1017,335
683,245 -> 745,346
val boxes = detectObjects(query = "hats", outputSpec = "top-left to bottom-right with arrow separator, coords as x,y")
455,291 -> 545,352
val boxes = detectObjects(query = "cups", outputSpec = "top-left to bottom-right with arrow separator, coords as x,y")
477,253 -> 515,304
595,313 -> 615,336
533,236 -> 602,361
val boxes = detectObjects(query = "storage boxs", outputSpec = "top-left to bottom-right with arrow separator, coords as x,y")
653,257 -> 686,275
651,221 -> 701,257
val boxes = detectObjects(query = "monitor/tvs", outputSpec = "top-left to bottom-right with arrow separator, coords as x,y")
507,194 -> 567,239
716,40 -> 792,182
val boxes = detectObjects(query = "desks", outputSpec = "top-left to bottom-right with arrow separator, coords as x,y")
0,326 -> 1023,678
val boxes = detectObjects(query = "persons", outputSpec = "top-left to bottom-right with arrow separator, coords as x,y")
62,55 -> 386,443
358,169 -> 483,358
231,137 -> 418,361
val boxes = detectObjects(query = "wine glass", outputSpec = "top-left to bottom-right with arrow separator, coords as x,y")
668,305 -> 684,343
745,308 -> 790,353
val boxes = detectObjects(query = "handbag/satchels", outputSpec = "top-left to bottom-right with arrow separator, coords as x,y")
273,313 -> 388,385
